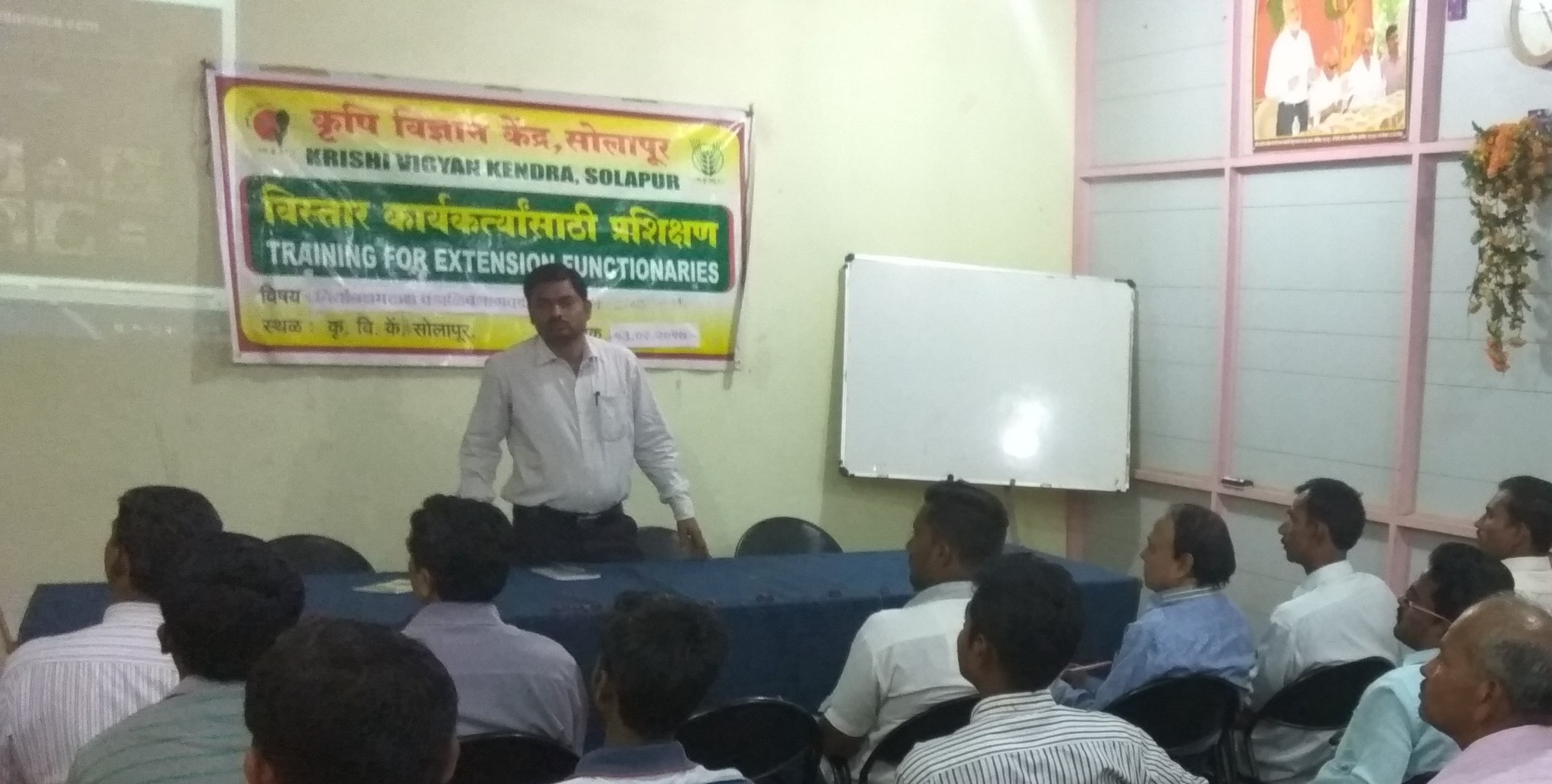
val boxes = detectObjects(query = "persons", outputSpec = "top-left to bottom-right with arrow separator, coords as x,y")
0,486 -> 755,784
818,477 -> 1552,784
456,262 -> 708,558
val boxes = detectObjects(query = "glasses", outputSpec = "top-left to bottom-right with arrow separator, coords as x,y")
1403,584 -> 1453,624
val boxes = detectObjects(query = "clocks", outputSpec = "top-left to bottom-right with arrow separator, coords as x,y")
1498,0 -> 1552,71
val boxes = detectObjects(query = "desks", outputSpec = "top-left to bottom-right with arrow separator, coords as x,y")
19,551 -> 1144,743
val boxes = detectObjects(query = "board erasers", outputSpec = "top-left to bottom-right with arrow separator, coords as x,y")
1221,476 -> 1254,490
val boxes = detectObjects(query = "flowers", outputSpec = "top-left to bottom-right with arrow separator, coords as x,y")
1458,109 -> 1552,375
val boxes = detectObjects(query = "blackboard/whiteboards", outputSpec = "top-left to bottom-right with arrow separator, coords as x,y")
838,254 -> 1138,497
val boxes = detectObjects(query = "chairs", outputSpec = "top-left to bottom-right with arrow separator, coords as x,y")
857,694 -> 981,784
688,695 -> 826,784
1101,669 -> 1242,784
641,526 -> 711,564
449,731 -> 580,784
1249,655 -> 1397,784
734,512 -> 847,557
266,534 -> 385,576
